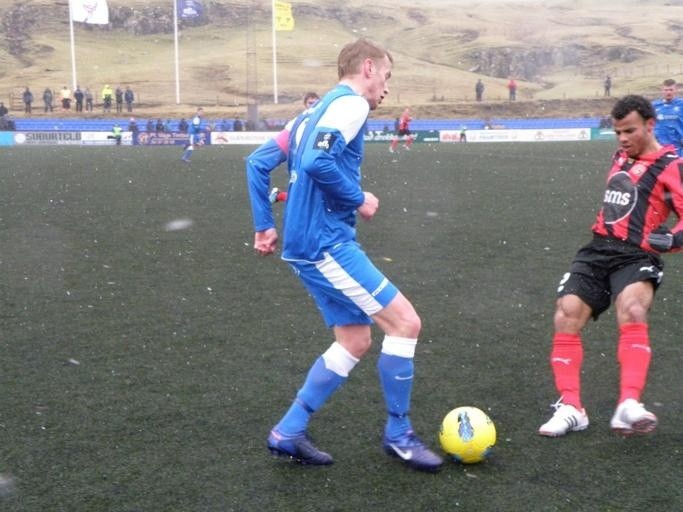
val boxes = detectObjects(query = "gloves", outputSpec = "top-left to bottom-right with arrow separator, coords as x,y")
646,224 -> 674,254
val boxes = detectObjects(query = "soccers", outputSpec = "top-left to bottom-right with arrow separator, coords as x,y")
438,405 -> 496,463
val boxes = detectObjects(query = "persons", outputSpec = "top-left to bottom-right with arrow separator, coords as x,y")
475,79 -> 484,101
603,75 -> 612,97
246,40 -> 445,474
269,92 -> 320,202
389,106 -> 413,152
650,79 -> 683,159
539,95 -> 683,437
460,126 -> 467,144
508,77 -> 517,101
0,84 -> 293,163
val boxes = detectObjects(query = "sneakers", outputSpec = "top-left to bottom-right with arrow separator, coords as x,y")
383,427 -> 444,473
609,397 -> 658,437
539,395 -> 590,439
267,426 -> 333,464
268,187 -> 279,204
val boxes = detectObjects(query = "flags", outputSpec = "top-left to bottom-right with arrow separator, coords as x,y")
176,0 -> 203,19
273,0 -> 294,32
70,0 -> 109,24
